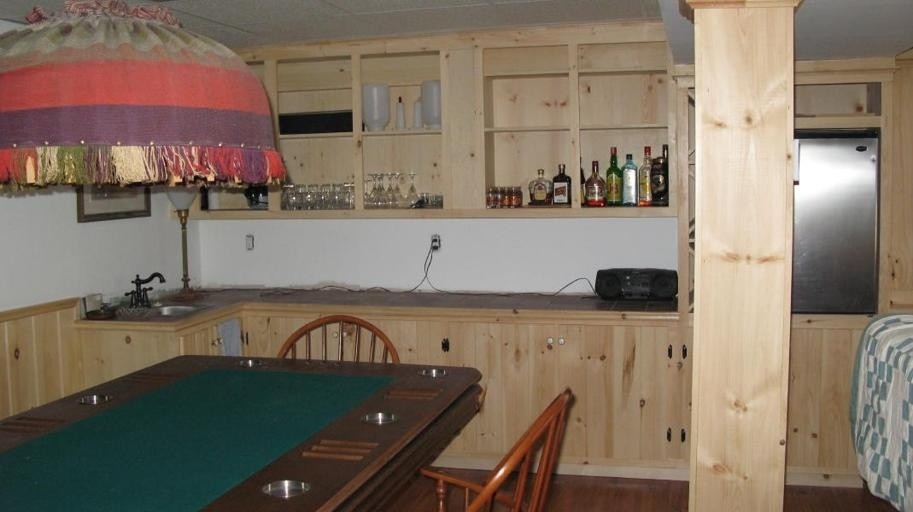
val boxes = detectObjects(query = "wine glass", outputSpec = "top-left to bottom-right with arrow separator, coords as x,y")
364,172 -> 418,209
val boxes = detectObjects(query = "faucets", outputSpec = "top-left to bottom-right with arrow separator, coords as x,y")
130,272 -> 165,305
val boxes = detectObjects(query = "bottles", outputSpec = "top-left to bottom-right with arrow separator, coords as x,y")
553,165 -> 571,205
413,100 -> 423,129
361,83 -> 391,133
529,167 -> 553,204
418,80 -> 440,130
486,186 -> 521,209
395,96 -> 405,129
581,144 -> 669,207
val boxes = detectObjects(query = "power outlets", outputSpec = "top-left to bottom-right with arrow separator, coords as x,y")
431,235 -> 440,251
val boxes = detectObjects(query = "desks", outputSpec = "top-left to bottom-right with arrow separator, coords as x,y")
1,354 -> 482,512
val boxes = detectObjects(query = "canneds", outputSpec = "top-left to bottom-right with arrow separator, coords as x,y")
487,186 -> 522,208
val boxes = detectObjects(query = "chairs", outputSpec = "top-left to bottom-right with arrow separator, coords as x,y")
279,317 -> 398,364
418,388 -> 577,512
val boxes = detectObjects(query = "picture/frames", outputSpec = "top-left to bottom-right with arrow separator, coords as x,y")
77,181 -> 151,224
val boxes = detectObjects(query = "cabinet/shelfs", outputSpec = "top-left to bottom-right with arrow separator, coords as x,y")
612,325 -> 692,467
485,41 -> 677,217
179,317 -> 243,356
199,51 -> 443,219
243,313 -> 418,366
418,319 -> 502,453
502,321 -> 613,462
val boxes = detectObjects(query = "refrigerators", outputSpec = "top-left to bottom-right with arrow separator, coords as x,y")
792,133 -> 881,316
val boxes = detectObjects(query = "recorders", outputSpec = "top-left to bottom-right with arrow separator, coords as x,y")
596,268 -> 678,301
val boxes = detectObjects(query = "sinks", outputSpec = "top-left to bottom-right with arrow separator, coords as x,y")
134,306 -> 202,321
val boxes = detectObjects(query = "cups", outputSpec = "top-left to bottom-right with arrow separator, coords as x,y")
281,183 -> 353,210
419,192 -> 442,208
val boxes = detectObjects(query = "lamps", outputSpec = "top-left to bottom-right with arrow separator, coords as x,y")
0,1 -> 289,196
